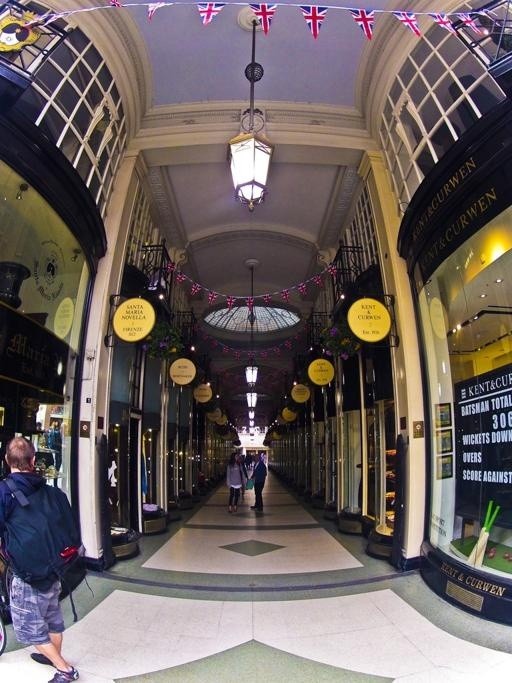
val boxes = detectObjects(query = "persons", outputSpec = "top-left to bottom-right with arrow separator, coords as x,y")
0,436 -> 82,683
250,454 -> 267,513
226,452 -> 247,513
36,421 -> 42,431
239,452 -> 267,500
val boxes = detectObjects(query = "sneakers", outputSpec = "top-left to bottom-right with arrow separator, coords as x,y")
48,666 -> 79,683
30,653 -> 56,665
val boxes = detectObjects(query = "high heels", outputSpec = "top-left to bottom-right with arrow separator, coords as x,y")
229,505 -> 237,513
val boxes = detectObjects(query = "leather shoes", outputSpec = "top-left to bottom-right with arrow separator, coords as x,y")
250,505 -> 263,511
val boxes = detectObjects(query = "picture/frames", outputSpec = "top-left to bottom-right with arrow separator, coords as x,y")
433,401 -> 453,479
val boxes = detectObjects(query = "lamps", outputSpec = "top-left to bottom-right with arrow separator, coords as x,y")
225,5 -> 276,212
242,256 -> 260,413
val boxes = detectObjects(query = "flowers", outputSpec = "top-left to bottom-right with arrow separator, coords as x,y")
316,325 -> 361,359
141,318 -> 183,361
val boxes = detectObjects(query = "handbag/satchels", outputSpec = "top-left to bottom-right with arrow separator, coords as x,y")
2,484 -> 86,593
246,479 -> 253,489
